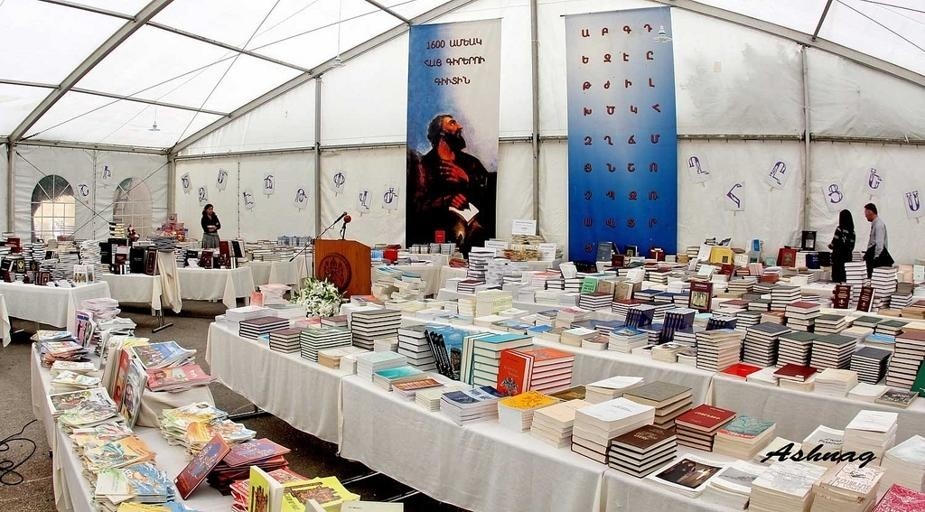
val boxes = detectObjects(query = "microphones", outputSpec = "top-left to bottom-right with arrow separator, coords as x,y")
340,216 -> 351,233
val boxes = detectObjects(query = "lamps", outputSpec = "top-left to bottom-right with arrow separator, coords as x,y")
655,0 -> 673,47
145,16 -> 161,139
332,1 -> 347,73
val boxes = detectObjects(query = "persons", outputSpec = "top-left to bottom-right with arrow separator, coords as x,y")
827,209 -> 857,282
863,203 -> 896,277
422,114 -> 492,247
200,204 -> 221,248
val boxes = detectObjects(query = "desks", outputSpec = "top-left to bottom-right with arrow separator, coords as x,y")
0,226 -> 925,510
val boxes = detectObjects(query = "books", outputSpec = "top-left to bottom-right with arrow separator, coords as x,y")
207,225 -> 215,229
